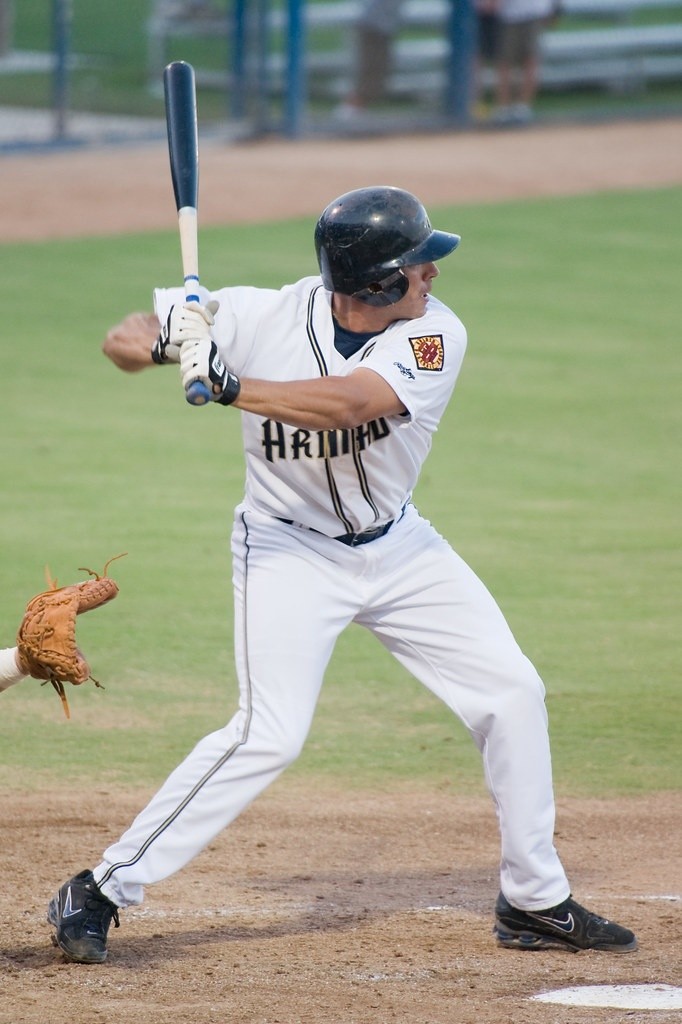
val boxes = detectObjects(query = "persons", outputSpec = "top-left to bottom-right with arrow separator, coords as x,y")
45,186 -> 637,962
473,0 -> 561,124
0,643 -> 30,693
331,0 -> 409,119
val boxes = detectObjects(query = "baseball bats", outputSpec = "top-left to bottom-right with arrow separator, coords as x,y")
163,61 -> 212,407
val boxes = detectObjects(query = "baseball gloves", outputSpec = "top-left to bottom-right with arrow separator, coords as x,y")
16,578 -> 118,686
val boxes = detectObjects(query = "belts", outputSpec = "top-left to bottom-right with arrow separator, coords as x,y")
276,506 -> 406,548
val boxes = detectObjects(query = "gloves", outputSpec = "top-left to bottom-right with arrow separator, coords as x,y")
156,300 -> 222,364
177,336 -> 240,409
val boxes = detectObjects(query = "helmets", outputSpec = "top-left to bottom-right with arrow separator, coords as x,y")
315,185 -> 461,305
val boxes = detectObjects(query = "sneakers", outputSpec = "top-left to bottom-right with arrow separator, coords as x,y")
494,891 -> 639,955
48,869 -> 120,963
